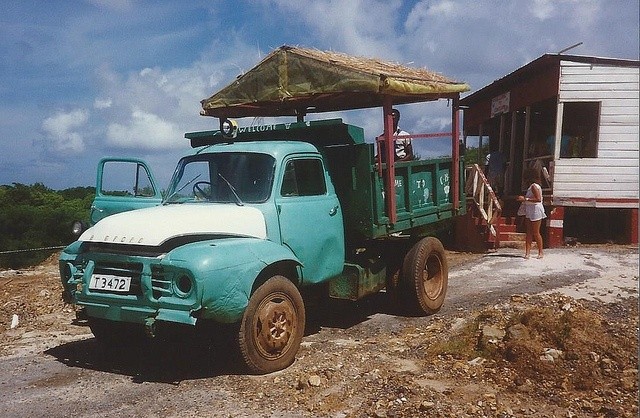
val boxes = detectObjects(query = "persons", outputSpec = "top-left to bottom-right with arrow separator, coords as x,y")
378,108 -> 413,161
516,166 -> 548,259
485,154 -> 491,176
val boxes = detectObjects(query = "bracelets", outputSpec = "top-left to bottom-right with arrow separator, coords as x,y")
526,197 -> 528,201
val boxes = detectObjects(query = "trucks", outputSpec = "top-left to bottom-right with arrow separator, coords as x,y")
59,118 -> 467,376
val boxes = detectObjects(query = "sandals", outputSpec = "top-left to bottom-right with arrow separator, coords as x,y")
525,253 -> 531,258
537,255 -> 543,258
